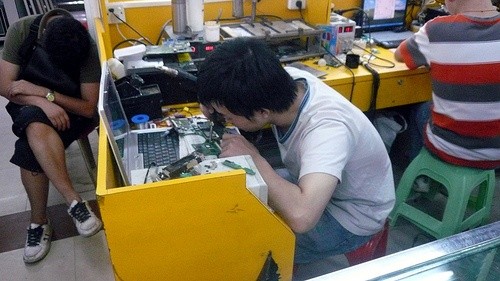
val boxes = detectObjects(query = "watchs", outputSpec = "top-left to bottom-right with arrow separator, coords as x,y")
46,89 -> 56,102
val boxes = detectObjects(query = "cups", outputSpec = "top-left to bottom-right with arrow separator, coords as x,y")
205,21 -> 221,43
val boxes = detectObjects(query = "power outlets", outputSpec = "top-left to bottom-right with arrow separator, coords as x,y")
106,2 -> 126,25
287,0 -> 306,9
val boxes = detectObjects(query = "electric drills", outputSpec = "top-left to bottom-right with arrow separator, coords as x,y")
154,66 -> 199,88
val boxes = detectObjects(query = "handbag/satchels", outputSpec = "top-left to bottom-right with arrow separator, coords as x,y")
370,109 -> 408,155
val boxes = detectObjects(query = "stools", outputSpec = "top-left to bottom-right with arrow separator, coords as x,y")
343,145 -> 496,266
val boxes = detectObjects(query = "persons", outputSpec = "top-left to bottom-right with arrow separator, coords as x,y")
0,8 -> 102,264
388,0 -> 500,196
194,37 -> 396,280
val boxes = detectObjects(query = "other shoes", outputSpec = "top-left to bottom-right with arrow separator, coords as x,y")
437,182 -> 449,196
412,175 -> 430,193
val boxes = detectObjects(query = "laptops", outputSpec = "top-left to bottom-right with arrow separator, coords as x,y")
97,62 -> 198,186
362,0 -> 417,49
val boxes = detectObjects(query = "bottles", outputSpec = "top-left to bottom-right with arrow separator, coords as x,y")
108,57 -> 126,79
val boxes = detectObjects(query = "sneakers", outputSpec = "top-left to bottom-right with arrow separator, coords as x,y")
23,217 -> 53,263
67,196 -> 103,239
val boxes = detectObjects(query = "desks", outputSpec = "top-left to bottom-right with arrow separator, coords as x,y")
96,0 -> 431,281
